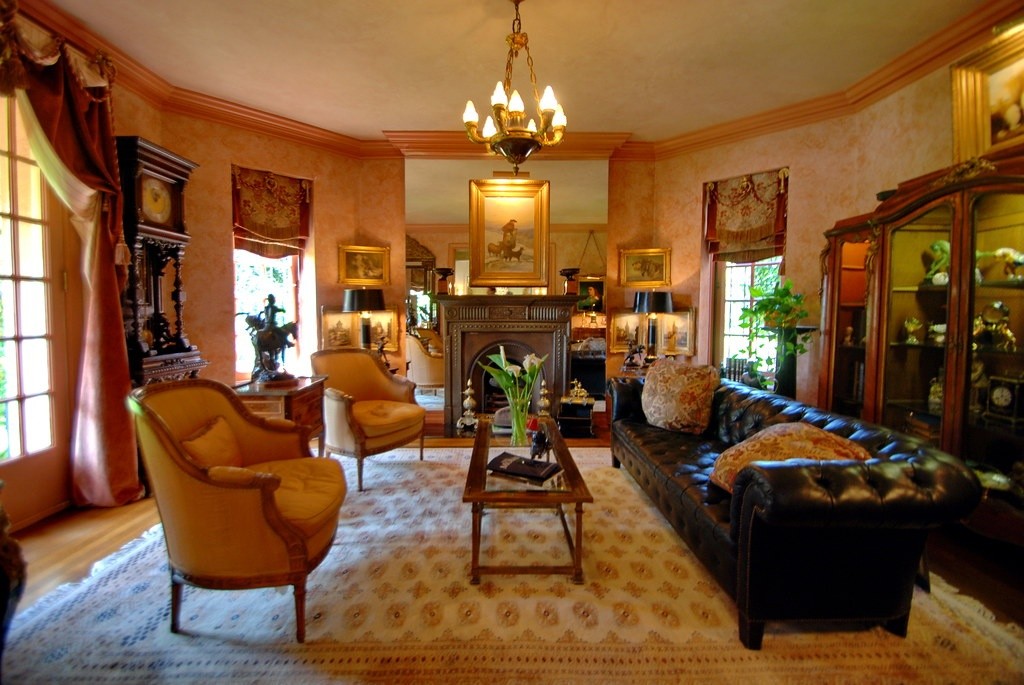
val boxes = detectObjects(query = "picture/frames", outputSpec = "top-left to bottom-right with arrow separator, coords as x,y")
618,246 -> 671,287
655,306 -> 695,357
470,178 -> 550,286
611,309 -> 647,352
577,276 -> 607,313
319,304 -> 402,361
336,244 -> 391,286
948,21 -> 1024,164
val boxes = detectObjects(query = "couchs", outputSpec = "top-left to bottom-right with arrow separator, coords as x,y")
606,378 -> 982,649
406,327 -> 444,388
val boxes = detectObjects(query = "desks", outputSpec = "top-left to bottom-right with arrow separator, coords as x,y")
228,375 -> 329,456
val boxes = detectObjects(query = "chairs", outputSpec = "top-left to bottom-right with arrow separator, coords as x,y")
124,379 -> 346,642
311,347 -> 425,491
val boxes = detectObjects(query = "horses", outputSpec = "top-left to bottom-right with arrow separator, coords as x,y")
257,320 -> 300,372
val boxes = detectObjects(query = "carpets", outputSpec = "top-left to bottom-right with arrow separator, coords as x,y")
0,440 -> 1023,685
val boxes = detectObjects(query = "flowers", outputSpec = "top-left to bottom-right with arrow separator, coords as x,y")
477,343 -> 550,402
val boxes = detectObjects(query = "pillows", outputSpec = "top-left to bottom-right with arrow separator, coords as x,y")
641,360 -> 721,435
709,420 -> 873,496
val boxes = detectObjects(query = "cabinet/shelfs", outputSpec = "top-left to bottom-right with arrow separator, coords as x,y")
828,268 -> 1024,485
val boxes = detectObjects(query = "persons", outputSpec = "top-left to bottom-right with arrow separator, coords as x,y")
374,336 -> 391,362
127,312 -> 173,388
259,293 -> 295,348
627,340 -> 634,352
407,303 -> 415,320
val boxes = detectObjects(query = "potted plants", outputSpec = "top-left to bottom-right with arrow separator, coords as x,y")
740,279 -> 814,389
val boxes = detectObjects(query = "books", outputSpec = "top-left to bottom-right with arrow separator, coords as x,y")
487,452 -> 565,487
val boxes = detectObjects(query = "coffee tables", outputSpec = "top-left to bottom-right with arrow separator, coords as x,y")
462,418 -> 594,585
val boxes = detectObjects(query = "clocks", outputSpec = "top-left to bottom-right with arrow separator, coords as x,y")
114,134 -> 212,495
983,376 -> 1020,431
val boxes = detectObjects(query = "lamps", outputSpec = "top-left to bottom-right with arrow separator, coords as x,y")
633,291 -> 674,364
462,0 -> 567,178
343,289 -> 386,350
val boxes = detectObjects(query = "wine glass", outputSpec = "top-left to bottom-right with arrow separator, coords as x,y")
905,320 -> 922,344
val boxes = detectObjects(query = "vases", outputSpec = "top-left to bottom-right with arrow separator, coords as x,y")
507,400 -> 529,447
